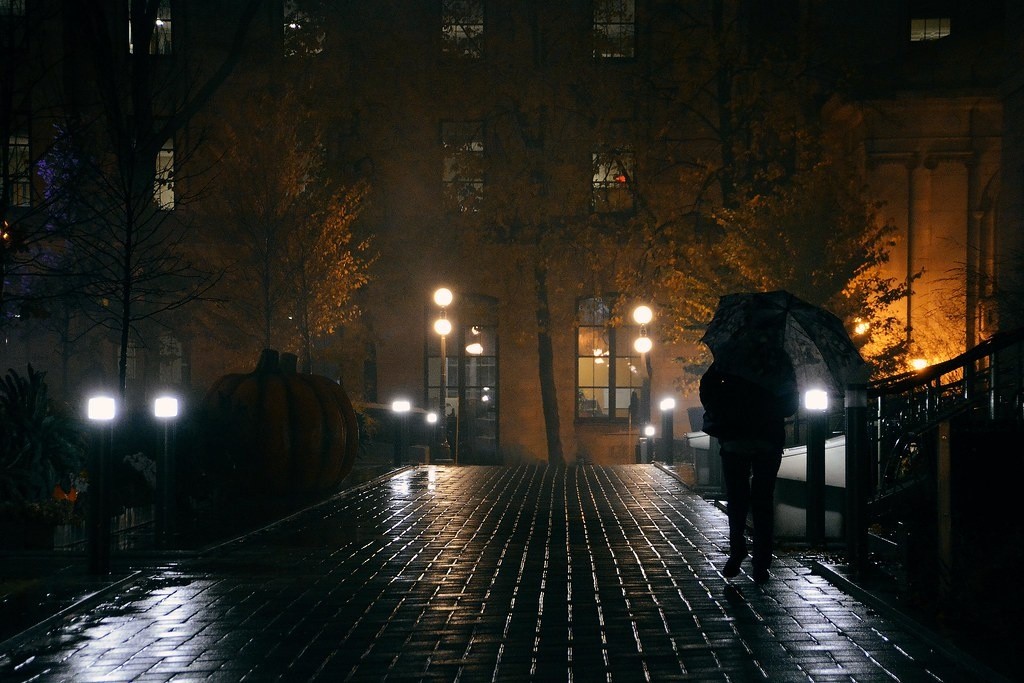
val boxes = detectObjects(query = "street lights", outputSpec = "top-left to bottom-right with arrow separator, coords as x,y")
633,306 -> 656,465
433,288 -> 453,459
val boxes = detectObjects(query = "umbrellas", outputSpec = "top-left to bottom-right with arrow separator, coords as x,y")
700,289 -> 864,394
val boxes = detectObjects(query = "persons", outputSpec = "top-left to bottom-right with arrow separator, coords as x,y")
699,299 -> 798,584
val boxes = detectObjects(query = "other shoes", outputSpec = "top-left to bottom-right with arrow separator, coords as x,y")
723,546 -> 748,578
752,568 -> 770,585
